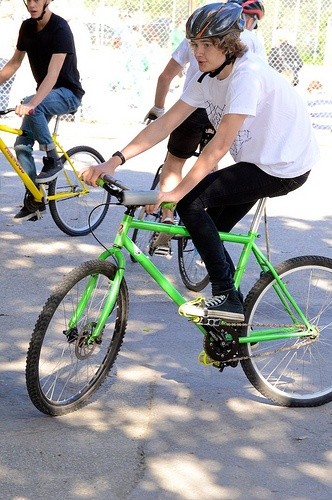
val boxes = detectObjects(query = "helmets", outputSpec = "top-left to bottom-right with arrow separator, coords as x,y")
186,2 -> 245,39
227,0 -> 265,20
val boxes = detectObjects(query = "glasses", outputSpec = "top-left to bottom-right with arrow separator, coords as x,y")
248,14 -> 258,30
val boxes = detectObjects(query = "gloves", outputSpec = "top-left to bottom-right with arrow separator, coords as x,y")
144,106 -> 165,125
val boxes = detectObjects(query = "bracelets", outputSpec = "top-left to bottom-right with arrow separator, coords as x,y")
112,151 -> 126,165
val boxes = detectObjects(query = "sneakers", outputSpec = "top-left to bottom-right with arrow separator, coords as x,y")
200,325 -> 259,363
13,196 -> 46,223
180,291 -> 245,323
35,156 -> 64,184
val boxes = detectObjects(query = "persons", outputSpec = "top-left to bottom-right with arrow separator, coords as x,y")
78,4 -> 318,319
143,0 -> 266,247
0,0 -> 85,221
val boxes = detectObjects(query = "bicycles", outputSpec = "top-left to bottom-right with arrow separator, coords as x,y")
0,107 -> 113,237
25,174 -> 332,418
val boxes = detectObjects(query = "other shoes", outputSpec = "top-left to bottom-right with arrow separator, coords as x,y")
148,217 -> 175,257
